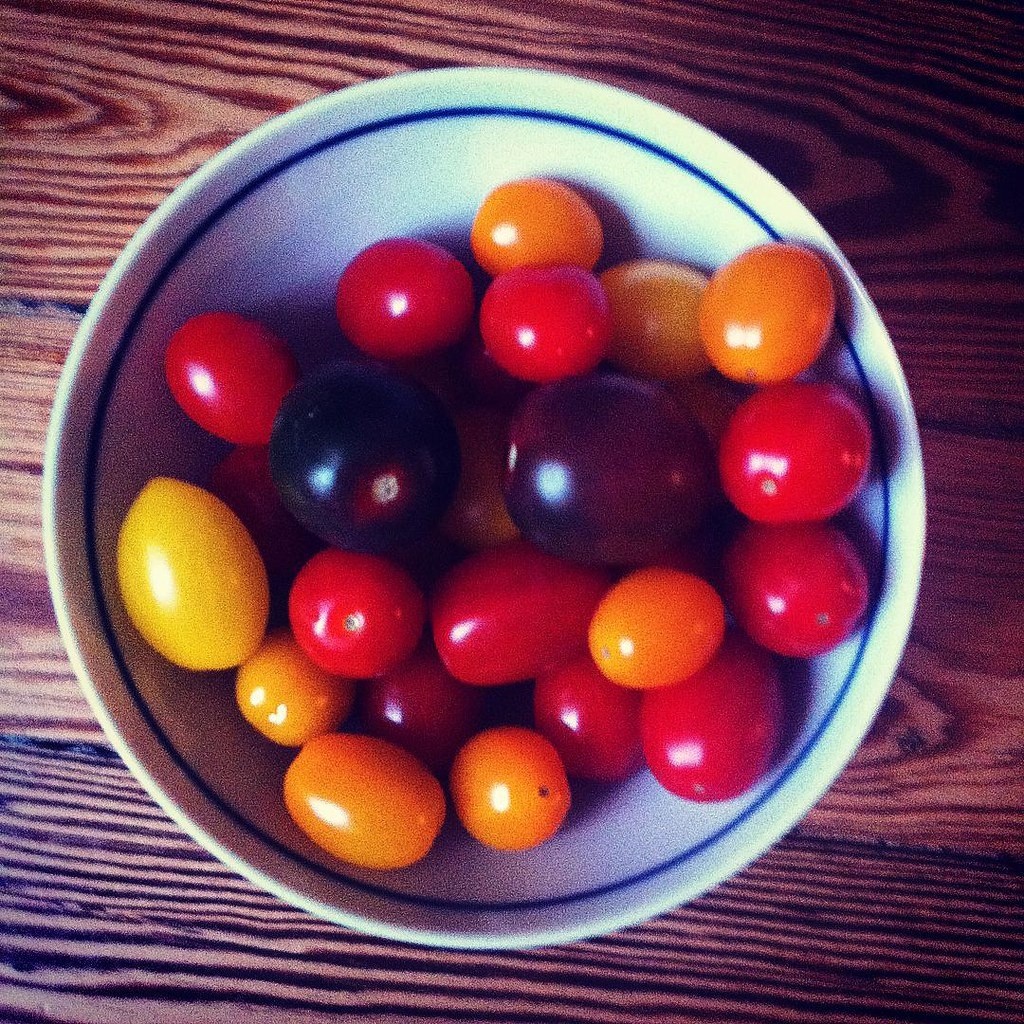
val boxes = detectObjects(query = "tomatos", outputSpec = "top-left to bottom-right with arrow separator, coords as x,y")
111,178 -> 869,870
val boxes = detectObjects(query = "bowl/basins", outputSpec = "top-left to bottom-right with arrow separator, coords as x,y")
38,66 -> 928,948
